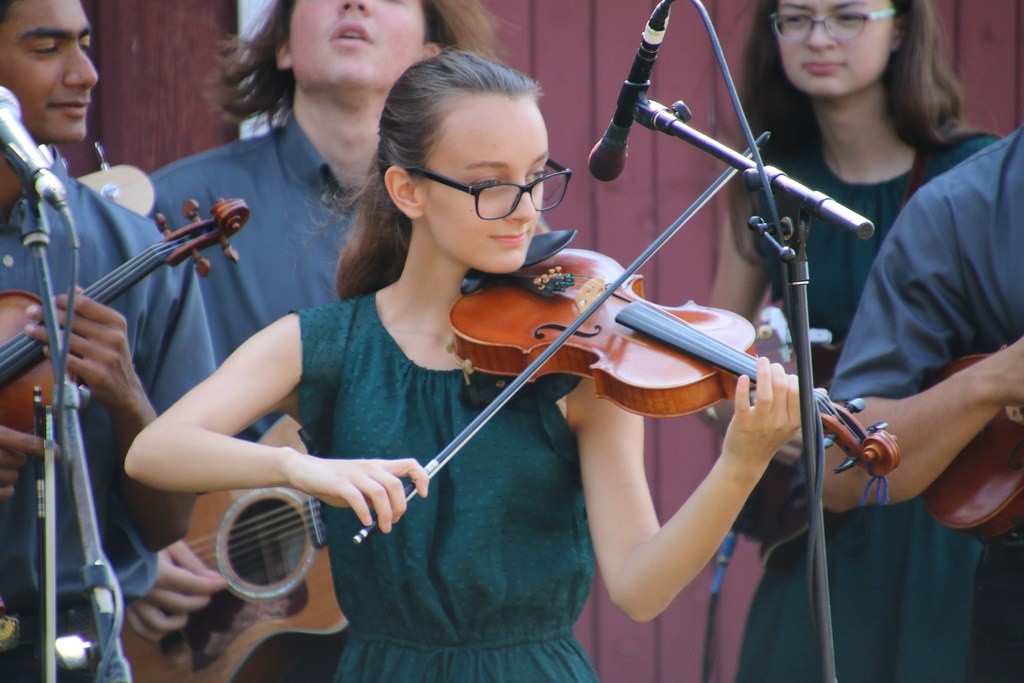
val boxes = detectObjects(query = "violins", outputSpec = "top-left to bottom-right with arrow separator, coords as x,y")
446,245 -> 900,486
1,195 -> 250,448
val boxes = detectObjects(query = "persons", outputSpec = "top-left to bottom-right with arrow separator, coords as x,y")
822,127 -> 1023,683
124,1 -> 801,683
712,0 -> 1001,683
0,0 -> 217,683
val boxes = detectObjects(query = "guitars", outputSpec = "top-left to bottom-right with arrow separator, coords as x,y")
86,403 -> 352,682
912,325 -> 1022,542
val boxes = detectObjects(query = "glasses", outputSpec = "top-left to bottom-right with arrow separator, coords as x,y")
769,8 -> 897,41
409,155 -> 571,221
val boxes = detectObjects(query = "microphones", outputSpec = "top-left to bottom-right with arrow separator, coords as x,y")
589,0 -> 674,183
0,86 -> 69,210
